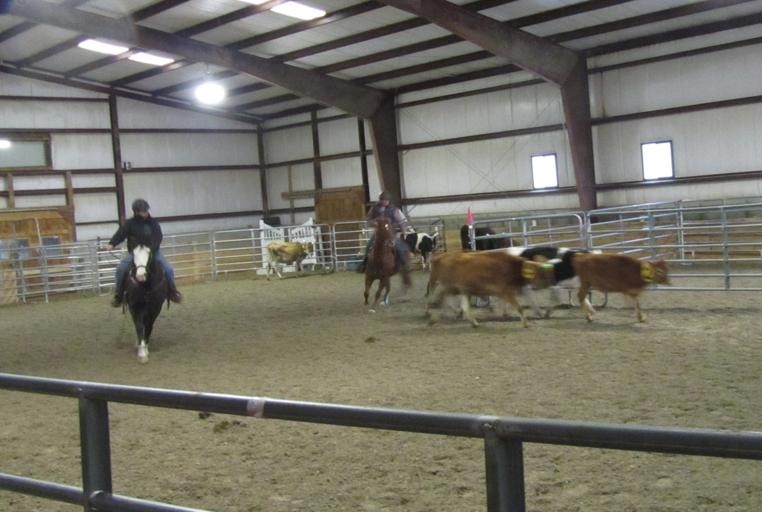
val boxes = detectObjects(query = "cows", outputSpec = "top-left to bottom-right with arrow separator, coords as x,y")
459,225 -> 519,252
424,244 -> 672,327
264,241 -> 315,281
400,231 -> 440,272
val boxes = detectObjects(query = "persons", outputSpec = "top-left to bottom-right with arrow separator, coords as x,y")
106,198 -> 184,309
355,189 -> 416,275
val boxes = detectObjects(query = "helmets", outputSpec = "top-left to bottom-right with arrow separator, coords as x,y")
131,199 -> 150,210
379,191 -> 393,200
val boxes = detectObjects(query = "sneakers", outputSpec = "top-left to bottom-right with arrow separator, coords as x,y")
111,293 -> 122,308
167,289 -> 183,304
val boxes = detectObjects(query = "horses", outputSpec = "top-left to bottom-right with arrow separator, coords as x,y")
122,235 -> 168,364
360,216 -> 412,314
